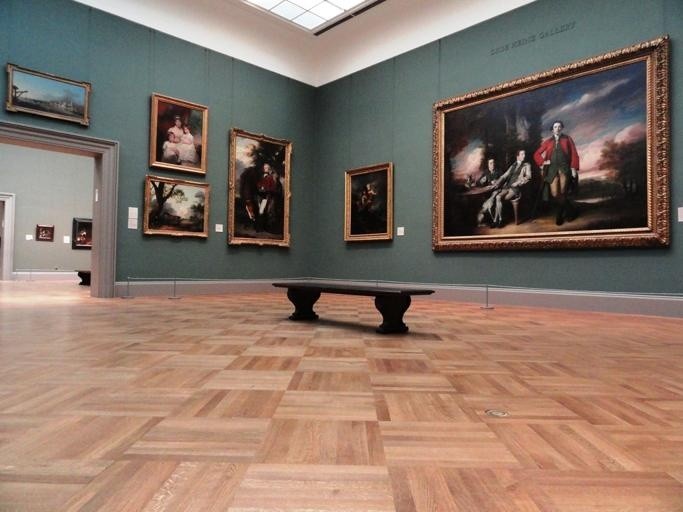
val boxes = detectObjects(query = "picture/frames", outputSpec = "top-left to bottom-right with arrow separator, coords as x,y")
4,59 -> 91,128
34,223 -> 54,242
424,38 -> 669,251
226,126 -> 294,249
343,161 -> 393,242
71,217 -> 92,250
149,89 -> 208,176
142,174 -> 209,239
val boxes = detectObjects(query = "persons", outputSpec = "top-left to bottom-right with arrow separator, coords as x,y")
236,138 -> 276,235
477,120 -> 580,228
361,183 -> 377,205
162,116 -> 199,164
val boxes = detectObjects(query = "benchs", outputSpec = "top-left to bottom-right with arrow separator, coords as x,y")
73,269 -> 90,286
272,278 -> 438,336
